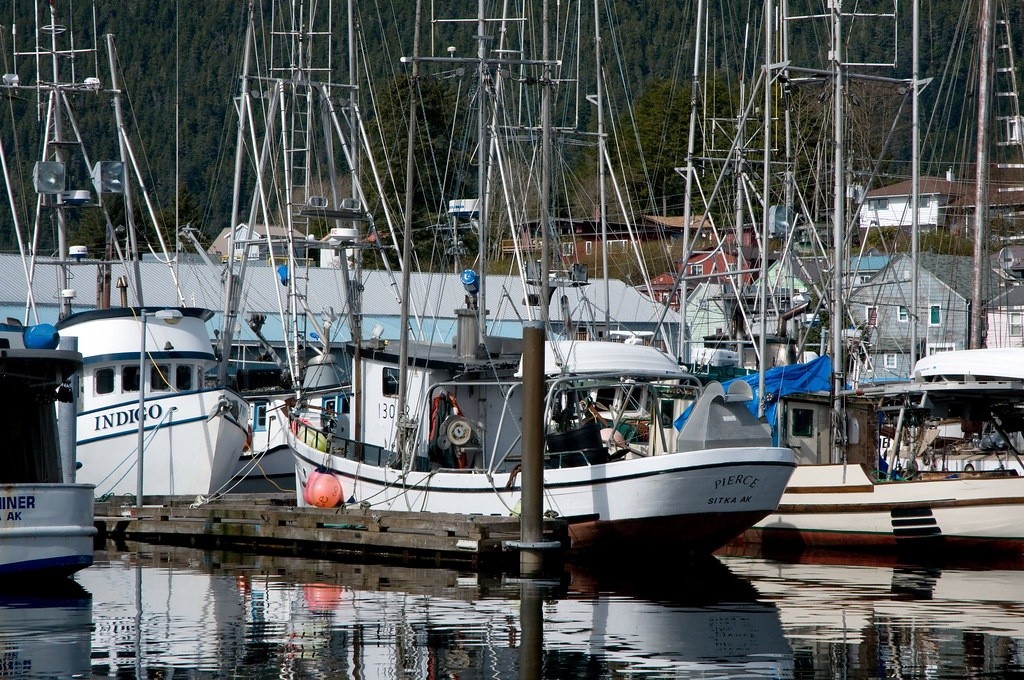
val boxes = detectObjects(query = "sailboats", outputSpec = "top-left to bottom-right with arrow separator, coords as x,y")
0,1 -> 1024,583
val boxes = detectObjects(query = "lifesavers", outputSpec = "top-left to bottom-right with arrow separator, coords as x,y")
243,424 -> 252,452
